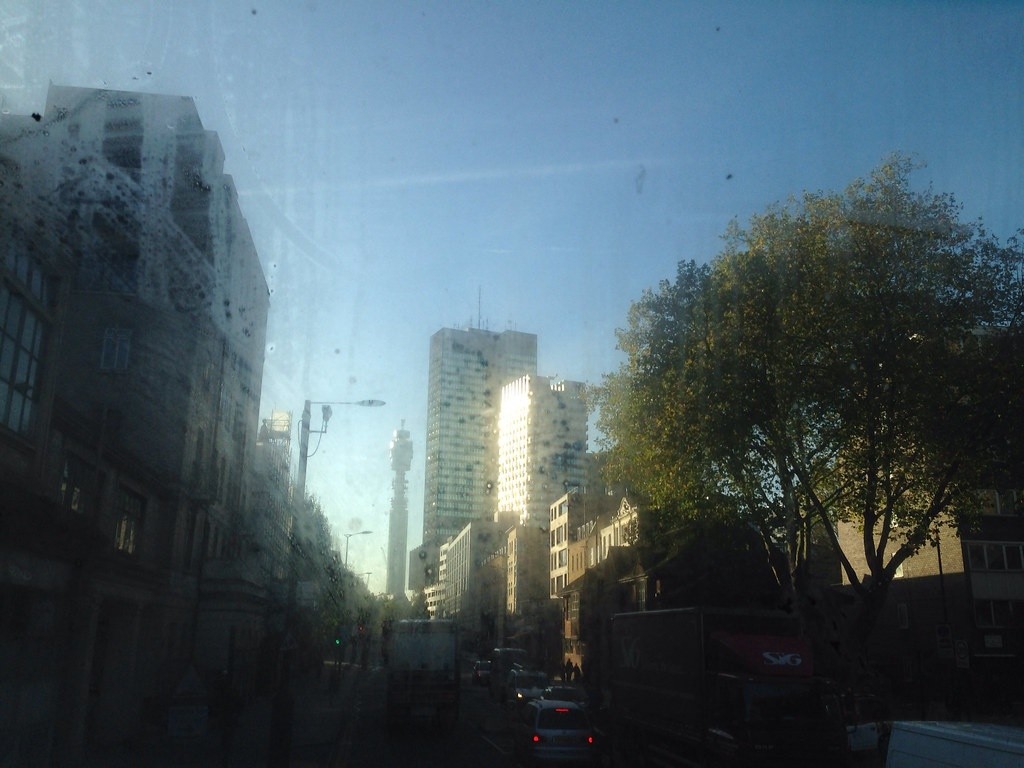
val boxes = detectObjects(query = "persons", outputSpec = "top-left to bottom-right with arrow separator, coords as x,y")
334,653 -> 343,665
315,655 -> 324,678
206,664 -> 241,768
559,658 -> 580,682
267,676 -> 295,768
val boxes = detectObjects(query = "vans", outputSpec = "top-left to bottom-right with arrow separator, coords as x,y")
472,646 -> 598,768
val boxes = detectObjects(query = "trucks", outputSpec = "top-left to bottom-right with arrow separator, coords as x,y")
608,604 -> 875,768
383,616 -> 461,727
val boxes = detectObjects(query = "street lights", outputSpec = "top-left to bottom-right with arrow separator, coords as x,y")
269,399 -> 387,768
344,529 -> 374,573
